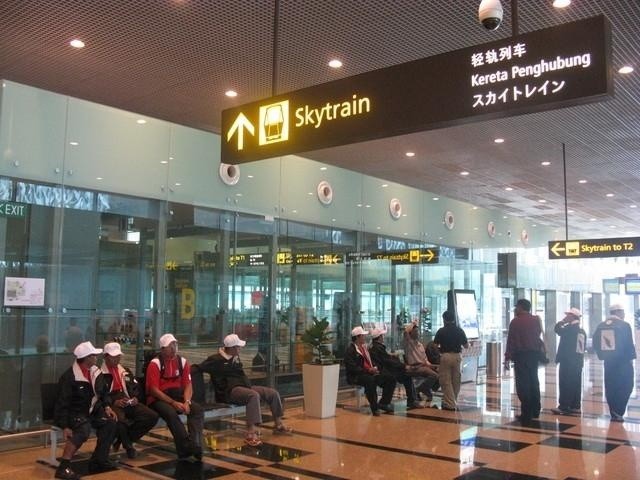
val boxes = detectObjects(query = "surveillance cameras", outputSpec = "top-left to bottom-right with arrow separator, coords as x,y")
477,0 -> 505,32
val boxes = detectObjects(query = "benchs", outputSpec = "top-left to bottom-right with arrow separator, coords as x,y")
339,367 -> 426,410
39,377 -> 246,467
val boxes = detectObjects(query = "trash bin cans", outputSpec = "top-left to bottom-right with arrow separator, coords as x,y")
486,342 -> 501,378
485,379 -> 503,413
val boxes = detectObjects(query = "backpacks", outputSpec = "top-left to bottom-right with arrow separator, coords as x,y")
141,349 -> 183,380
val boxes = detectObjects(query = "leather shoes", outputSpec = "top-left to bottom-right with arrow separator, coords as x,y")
55,466 -> 80,480
88,457 -> 113,472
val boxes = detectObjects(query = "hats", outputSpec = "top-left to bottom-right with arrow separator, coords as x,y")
159,333 -> 178,349
564,307 -> 582,321
351,326 -> 369,337
73,341 -> 104,360
223,334 -> 246,348
514,299 -> 531,311
608,303 -> 624,311
371,329 -> 386,339
102,342 -> 124,357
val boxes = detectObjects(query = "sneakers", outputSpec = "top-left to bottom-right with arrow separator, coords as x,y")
378,402 -> 394,412
407,384 -> 432,409
176,455 -> 202,465
273,422 -> 293,434
127,448 -> 137,459
113,440 -> 121,452
244,431 -> 263,447
373,410 -> 380,416
514,407 -> 624,422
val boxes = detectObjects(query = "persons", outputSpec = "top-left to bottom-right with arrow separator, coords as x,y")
550,308 -> 587,414
198,334 -> 293,447
100,342 -> 159,459
345,310 -> 470,417
504,299 -> 542,421
53,341 -> 118,480
592,304 -> 637,422
145,333 -> 204,463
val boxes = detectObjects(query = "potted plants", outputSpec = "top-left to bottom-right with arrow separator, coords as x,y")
301,315 -> 341,419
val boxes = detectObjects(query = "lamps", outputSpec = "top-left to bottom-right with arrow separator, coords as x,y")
479,0 -> 504,31
